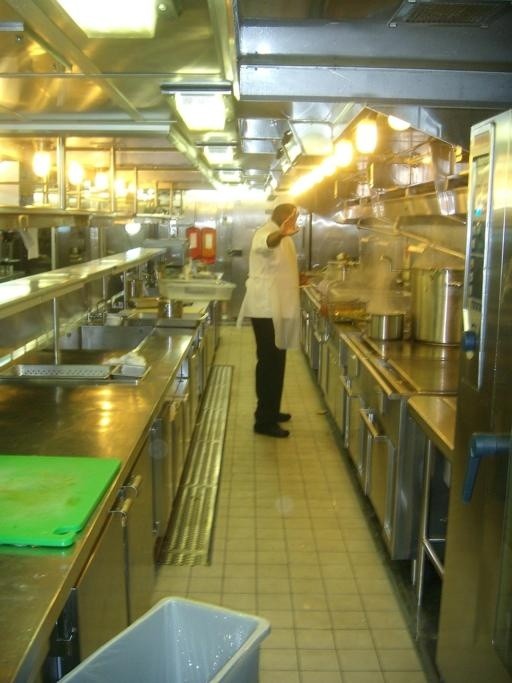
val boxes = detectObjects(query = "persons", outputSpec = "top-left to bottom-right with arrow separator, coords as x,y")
236,202 -> 302,438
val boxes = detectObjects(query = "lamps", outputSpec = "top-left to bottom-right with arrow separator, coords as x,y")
54,0 -> 159,40
195,143 -> 237,165
217,169 -> 241,183
164,83 -> 231,130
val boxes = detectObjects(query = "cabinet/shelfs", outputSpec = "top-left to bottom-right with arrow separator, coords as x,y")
69,301 -> 223,670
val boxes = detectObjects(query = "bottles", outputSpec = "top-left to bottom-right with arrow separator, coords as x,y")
336,262 -> 345,281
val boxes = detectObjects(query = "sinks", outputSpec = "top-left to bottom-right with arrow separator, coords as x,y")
40,324 -> 156,353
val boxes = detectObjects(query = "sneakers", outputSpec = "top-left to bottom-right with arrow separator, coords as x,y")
254,412 -> 291,437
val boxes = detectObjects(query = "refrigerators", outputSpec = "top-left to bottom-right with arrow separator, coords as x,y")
431,109 -> 512,683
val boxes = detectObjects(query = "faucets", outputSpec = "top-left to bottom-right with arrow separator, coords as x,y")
96,296 -> 113,313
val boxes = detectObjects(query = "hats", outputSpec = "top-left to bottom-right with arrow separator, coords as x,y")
265,195 -> 298,214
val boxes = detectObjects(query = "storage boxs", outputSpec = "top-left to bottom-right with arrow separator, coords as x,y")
56,597 -> 271,683
157,278 -> 237,300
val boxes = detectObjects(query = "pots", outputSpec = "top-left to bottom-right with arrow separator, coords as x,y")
411,264 -> 465,346
158,299 -> 193,320
360,311 -> 404,341
